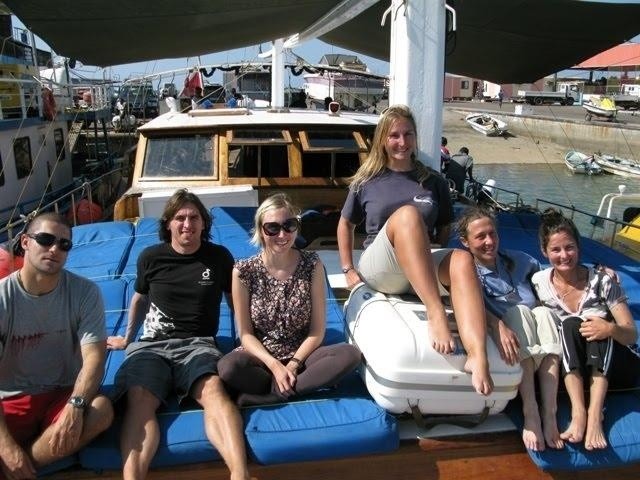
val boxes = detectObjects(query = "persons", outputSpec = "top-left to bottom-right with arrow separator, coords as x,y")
116,98 -> 128,130
444,146 -> 474,193
104,189 -> 258,479
452,206 -> 568,452
190,86 -> 332,111
216,193 -> 362,405
532,210 -> 636,450
336,102 -> 495,398
441,136 -> 450,168
0,210 -> 116,478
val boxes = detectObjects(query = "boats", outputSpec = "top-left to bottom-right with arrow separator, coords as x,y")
0,5 -> 131,249
588,184 -> 640,265
465,112 -> 508,137
565,151 -> 601,175
583,99 -> 619,121
302,54 -> 387,111
0,0 -> 640,480
594,152 -> 640,179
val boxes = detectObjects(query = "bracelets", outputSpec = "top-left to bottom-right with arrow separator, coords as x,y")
342,265 -> 354,273
291,357 -> 303,369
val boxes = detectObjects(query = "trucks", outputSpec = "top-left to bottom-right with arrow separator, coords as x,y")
600,84 -> 640,109
518,82 -> 580,106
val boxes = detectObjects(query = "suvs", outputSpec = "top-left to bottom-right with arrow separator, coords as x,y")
113,77 -> 160,120
156,83 -> 177,99
202,84 -> 227,103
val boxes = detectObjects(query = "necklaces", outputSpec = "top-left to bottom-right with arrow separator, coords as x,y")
555,270 -> 583,297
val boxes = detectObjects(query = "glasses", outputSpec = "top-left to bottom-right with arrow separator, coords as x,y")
28,234 -> 72,250
261,218 -> 298,236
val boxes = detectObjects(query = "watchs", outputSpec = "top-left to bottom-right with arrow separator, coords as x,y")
68,396 -> 89,409
